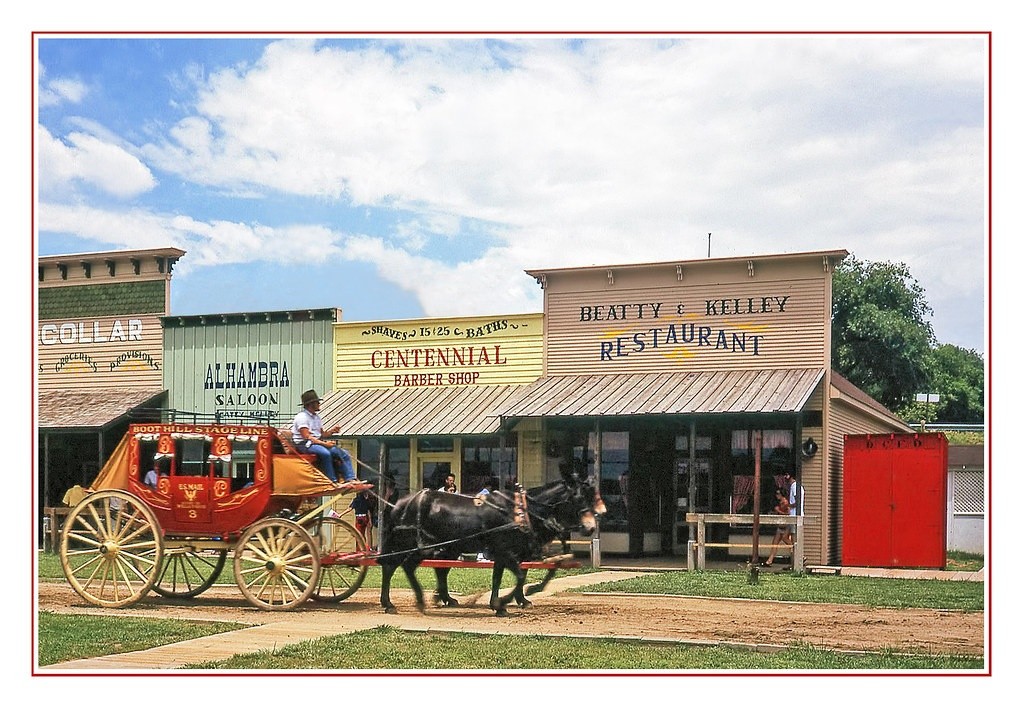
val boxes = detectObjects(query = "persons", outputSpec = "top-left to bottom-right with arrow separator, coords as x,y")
62,477 -> 88,547
291,388 -> 368,485
783,470 -> 807,571
437,473 -> 459,494
339,482 -> 399,550
476,482 -> 493,561
144,462 -> 167,487
761,487 -> 793,566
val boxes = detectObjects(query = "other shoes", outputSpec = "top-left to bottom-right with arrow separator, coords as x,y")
760,562 -> 772,567
344,479 -> 367,484
335,482 -> 347,486
803,556 -> 807,563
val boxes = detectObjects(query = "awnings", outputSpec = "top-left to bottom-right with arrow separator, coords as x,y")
281,384 -> 532,438
489,369 -> 823,415
38,391 -> 165,429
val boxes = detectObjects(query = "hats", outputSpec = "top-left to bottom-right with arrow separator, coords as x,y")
297,390 -> 323,406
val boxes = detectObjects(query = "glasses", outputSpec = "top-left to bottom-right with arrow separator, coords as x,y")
776,493 -> 781,495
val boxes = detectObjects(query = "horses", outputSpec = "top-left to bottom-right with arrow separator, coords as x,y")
379,472 -> 606,615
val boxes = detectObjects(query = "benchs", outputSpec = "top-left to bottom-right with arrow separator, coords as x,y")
273,429 -> 339,464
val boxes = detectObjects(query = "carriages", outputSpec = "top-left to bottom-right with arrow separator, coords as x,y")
58,405 -> 610,613
